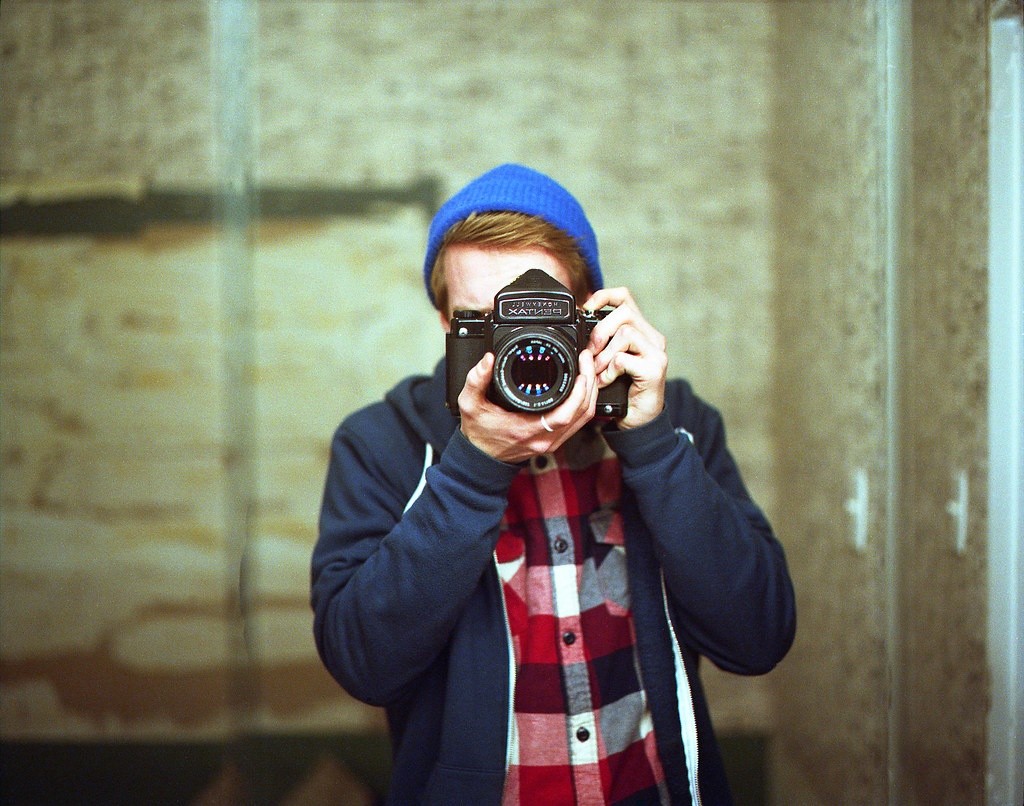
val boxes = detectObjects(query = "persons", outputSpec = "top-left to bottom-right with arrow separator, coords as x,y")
311,164 -> 797,806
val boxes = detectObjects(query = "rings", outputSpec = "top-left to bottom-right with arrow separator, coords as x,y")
541,414 -> 554,432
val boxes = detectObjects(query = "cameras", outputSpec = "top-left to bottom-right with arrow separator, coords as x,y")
447,268 -> 629,420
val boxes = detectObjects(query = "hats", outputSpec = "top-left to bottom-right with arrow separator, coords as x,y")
423,162 -> 605,314
